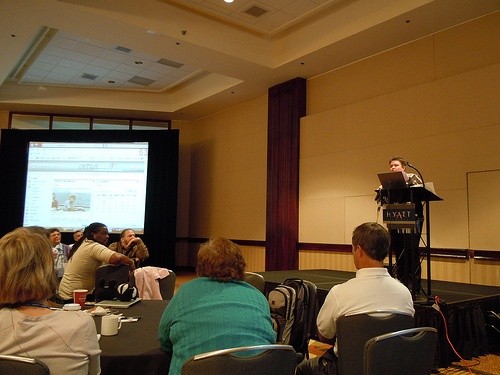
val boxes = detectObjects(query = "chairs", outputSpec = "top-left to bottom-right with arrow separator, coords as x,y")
0,257 -> 448,375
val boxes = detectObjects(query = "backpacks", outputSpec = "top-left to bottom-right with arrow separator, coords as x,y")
267,278 -> 309,350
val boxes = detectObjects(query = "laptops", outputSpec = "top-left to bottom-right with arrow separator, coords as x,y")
377,171 -> 406,189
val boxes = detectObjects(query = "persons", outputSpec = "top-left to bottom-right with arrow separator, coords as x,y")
295,221 -> 415,375
52,192 -> 58,209
157,235 -> 277,375
58,221 -> 136,305
375,156 -> 424,299
0,226 -> 102,375
63,195 -> 78,211
28,225 -> 150,271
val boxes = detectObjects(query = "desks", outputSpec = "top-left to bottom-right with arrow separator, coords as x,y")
78,299 -> 177,375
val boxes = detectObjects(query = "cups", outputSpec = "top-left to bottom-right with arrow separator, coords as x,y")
73,289 -> 88,308
101,316 -> 122,336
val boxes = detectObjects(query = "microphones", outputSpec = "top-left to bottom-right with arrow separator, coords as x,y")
408,164 -> 425,188
